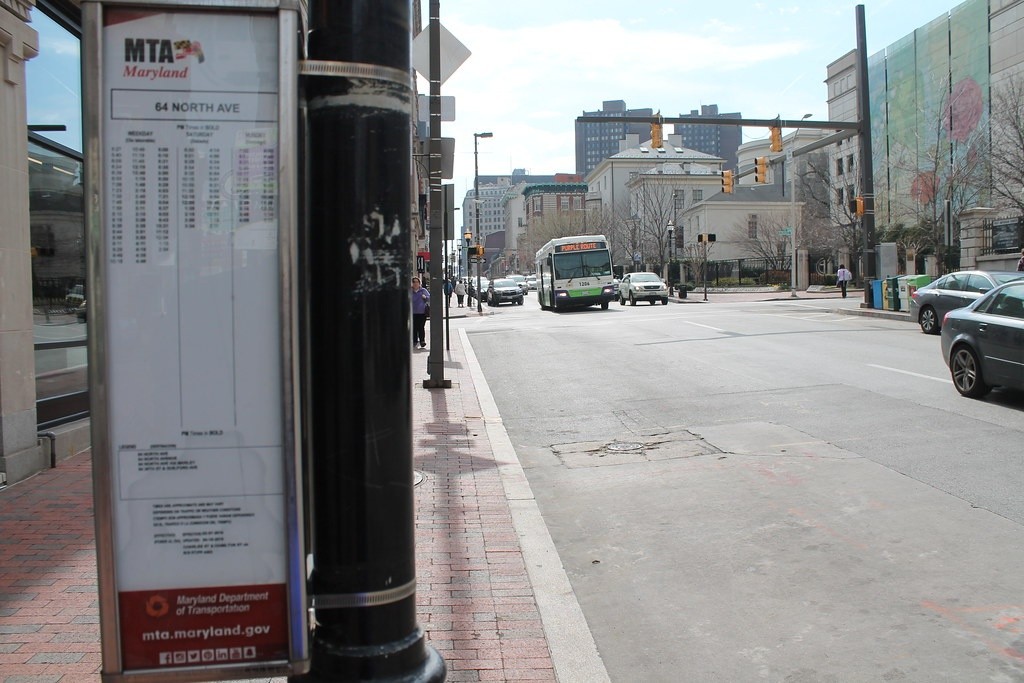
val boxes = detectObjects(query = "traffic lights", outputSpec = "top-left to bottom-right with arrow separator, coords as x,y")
849,200 -> 863,213
698,234 -> 703,242
768,125 -> 783,152
754,157 -> 766,183
722,170 -> 733,193
708,234 -> 716,242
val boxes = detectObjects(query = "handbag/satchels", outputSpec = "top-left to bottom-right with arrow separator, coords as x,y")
835,277 -> 840,288
425,303 -> 430,317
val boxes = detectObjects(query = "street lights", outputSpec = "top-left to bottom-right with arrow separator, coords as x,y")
474,132 -> 494,312
791,112 -> 814,297
666,220 -> 675,297
457,244 -> 463,281
464,227 -> 473,307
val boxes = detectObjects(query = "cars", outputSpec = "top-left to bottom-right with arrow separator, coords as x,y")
505,274 -> 528,295
613,278 -> 620,301
65,284 -> 85,308
910,270 -> 1024,335
486,279 -> 524,307
941,278 -> 1024,400
526,276 -> 538,290
462,276 -> 490,302
74,300 -> 86,319
619,271 -> 668,307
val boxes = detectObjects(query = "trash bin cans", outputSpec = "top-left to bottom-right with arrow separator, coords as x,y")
873,274 -> 931,311
679,285 -> 687,298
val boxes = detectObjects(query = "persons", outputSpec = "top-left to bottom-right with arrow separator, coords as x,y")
412,276 -> 430,348
1016,248 -> 1024,271
444,279 -> 453,307
837,264 -> 848,297
463,278 -> 467,293
455,280 -> 465,307
466,282 -> 472,307
451,276 -> 456,292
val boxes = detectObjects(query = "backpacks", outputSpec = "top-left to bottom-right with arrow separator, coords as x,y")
844,269 -> 852,282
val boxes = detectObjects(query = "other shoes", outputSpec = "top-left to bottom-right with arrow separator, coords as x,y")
413,341 -> 419,347
420,342 -> 426,347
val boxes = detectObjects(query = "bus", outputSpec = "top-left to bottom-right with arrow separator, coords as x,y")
535,234 -> 615,312
